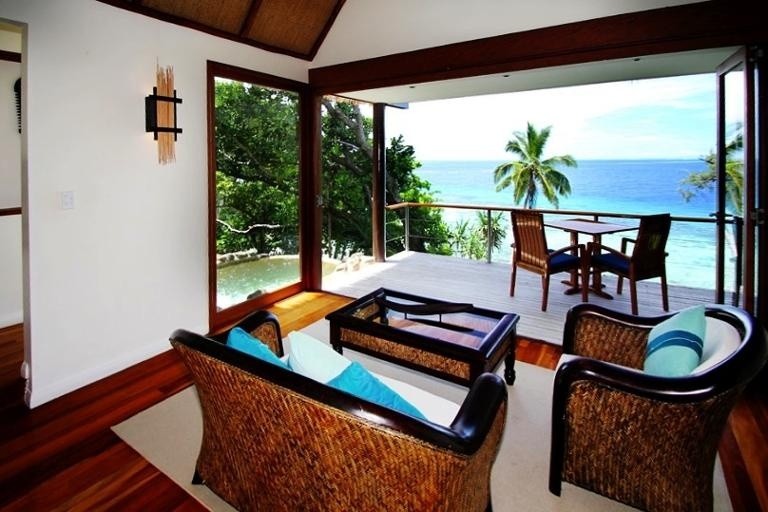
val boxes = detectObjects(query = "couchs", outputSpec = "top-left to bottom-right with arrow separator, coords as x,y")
170,303 -> 510,509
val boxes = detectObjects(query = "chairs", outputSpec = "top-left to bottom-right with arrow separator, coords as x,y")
550,303 -> 766,511
510,208 -> 671,316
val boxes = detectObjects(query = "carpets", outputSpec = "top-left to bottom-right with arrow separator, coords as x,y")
111,314 -> 732,510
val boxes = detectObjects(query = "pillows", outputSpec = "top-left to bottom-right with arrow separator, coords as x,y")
224,323 -> 428,423
639,305 -> 709,380
